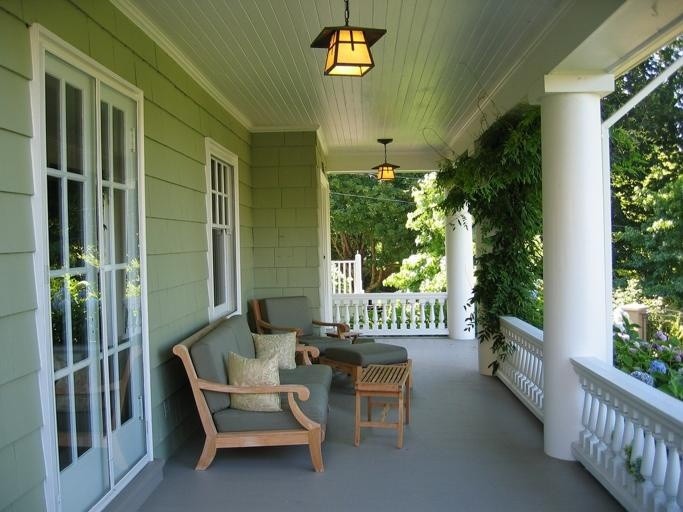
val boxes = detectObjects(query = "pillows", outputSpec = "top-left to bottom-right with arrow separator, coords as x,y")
54,358 -> 83,412
250,330 -> 298,370
227,351 -> 284,413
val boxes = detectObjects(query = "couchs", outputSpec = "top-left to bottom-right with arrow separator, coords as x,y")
173,314 -> 332,472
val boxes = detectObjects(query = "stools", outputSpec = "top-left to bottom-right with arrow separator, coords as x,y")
354,364 -> 411,449
320,342 -> 413,395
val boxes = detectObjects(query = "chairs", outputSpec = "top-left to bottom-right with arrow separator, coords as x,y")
54,339 -> 130,448
248,295 -> 353,376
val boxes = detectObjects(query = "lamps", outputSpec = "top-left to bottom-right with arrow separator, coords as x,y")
372,139 -> 401,182
310,0 -> 388,77
